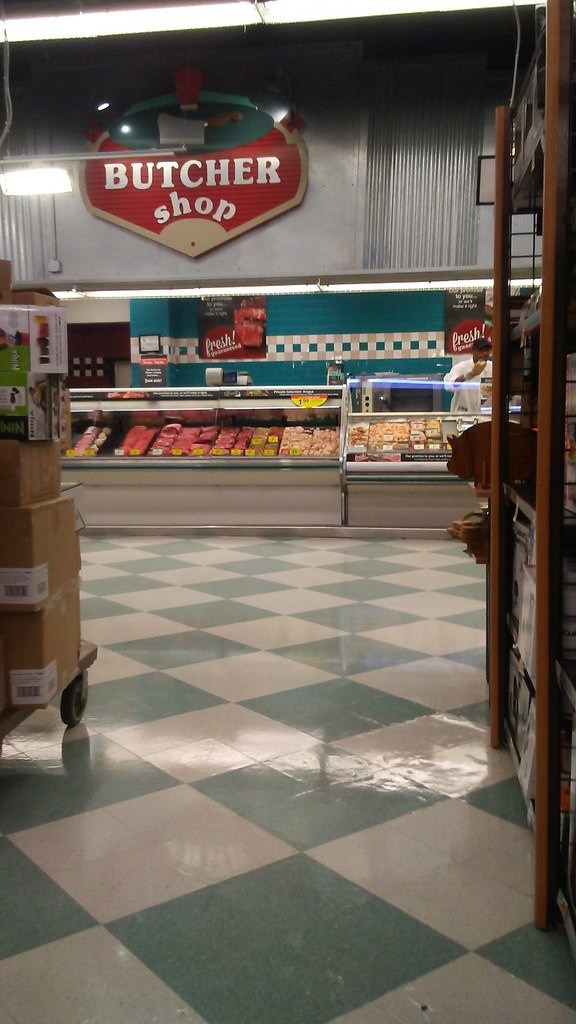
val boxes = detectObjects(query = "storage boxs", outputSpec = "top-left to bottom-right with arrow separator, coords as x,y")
0,574 -> 81,710
0,494 -> 81,613
0,372 -> 71,442
0,440 -> 61,507
0,304 -> 69,374
0,260 -> 59,306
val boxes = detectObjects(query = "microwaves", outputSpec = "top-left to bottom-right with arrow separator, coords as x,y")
362,375 -> 441,412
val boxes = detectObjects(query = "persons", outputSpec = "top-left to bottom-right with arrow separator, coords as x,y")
443,338 -> 492,412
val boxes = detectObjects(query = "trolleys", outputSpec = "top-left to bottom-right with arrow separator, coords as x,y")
0,637 -> 100,757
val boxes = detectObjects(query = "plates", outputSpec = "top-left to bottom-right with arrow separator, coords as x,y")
375,372 -> 399,377
